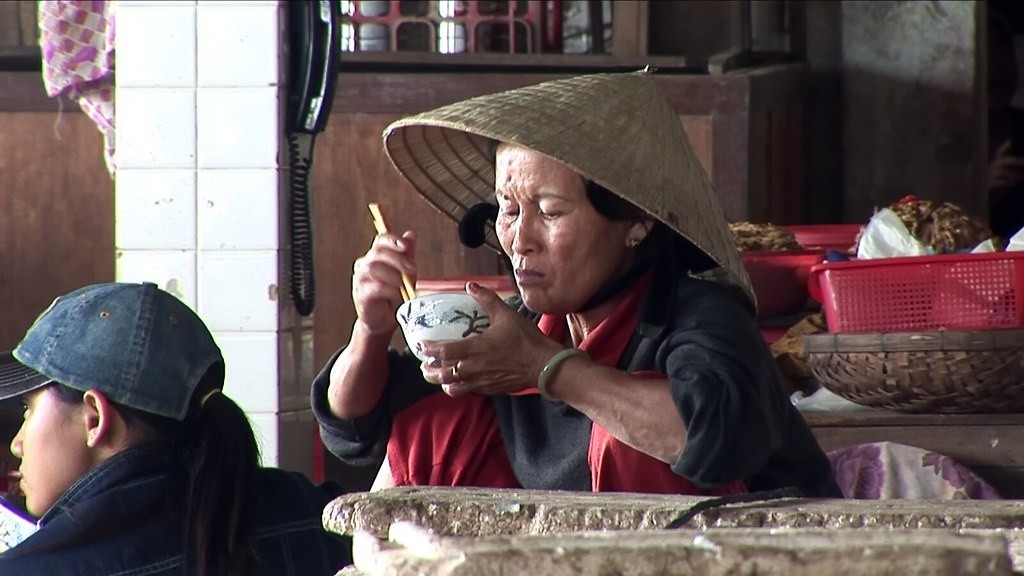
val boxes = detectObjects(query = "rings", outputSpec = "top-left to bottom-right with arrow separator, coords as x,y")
453,362 -> 460,379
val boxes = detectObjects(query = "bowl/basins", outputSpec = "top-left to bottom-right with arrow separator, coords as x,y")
396,294 -> 491,367
738,247 -> 826,313
784,225 -> 864,253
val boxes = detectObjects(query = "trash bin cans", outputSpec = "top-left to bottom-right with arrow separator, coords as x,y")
288,0 -> 342,136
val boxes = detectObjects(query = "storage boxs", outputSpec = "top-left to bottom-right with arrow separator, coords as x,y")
741,222 -> 1024,334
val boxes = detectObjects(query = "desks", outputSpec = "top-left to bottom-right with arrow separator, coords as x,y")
792,409 -> 1024,490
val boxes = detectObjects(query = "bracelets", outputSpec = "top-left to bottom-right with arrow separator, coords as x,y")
538,349 -> 591,401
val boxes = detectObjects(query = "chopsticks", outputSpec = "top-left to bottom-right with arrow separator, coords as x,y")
369,202 -> 415,301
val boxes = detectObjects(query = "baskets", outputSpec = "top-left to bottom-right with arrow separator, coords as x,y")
805,250 -> 1024,415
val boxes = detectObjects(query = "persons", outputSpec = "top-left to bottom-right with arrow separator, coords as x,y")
310,64 -> 843,495
0,282 -> 353,576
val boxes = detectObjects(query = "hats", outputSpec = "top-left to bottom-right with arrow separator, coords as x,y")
0,282 -> 224,420
382,64 -> 759,316
